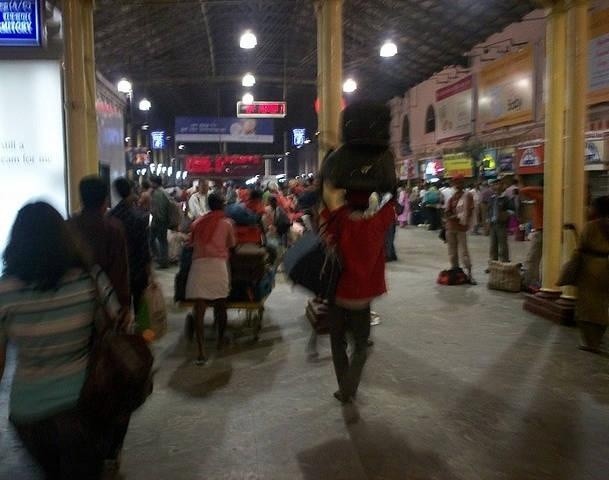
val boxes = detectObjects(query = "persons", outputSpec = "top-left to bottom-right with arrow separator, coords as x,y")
397,174 -> 519,283
563,196 -> 608,351
317,173 -> 395,400
0,170 -> 181,478
183,179 -> 318,363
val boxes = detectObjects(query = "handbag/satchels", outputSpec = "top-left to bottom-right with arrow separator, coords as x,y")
487,259 -> 523,292
305,297 -> 338,333
136,281 -> 168,341
437,267 -> 467,286
90,330 -> 154,419
273,205 -> 291,234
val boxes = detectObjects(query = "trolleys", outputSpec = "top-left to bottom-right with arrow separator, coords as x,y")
175,243 -> 270,346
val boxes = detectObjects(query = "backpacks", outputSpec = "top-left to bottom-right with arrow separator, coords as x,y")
167,201 -> 183,231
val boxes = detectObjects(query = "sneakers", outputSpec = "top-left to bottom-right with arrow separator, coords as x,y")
282,232 -> 337,299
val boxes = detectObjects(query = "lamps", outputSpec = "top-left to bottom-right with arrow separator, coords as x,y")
239,28 -> 257,49
139,97 -> 151,111
117,76 -> 131,94
242,71 -> 256,87
343,76 -> 357,93
241,92 -> 253,104
380,38 -> 398,59
